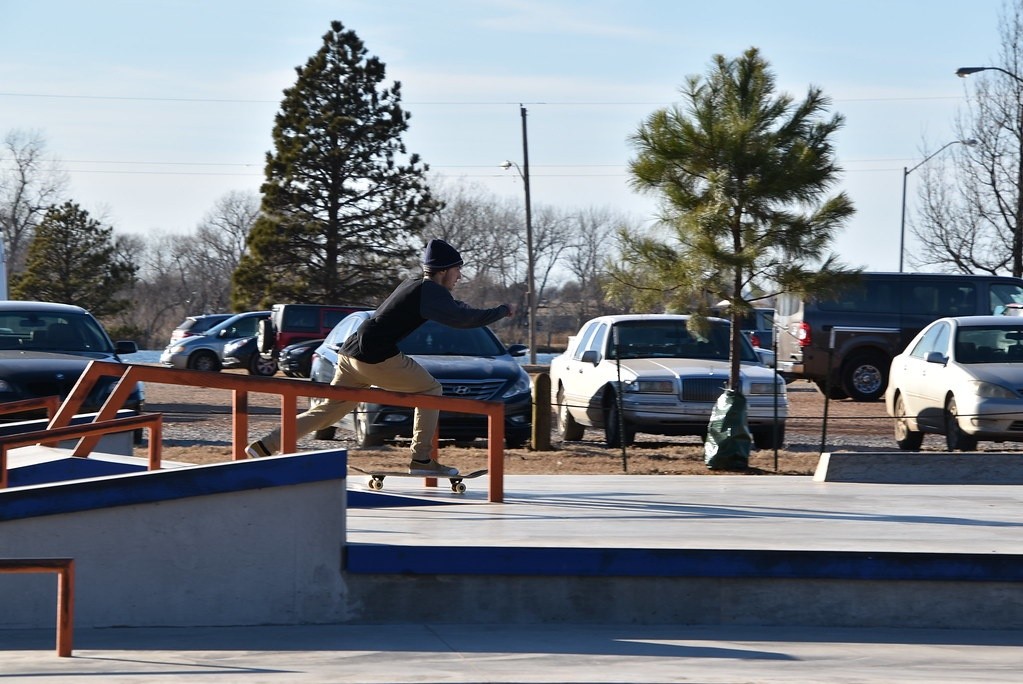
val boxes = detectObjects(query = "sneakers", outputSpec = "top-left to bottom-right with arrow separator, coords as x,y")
244,440 -> 272,458
409,459 -> 460,476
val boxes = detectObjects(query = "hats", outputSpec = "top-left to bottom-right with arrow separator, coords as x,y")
423,238 -> 464,272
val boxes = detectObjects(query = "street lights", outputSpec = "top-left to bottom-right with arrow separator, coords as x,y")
896,140 -> 978,270
500,108 -> 537,312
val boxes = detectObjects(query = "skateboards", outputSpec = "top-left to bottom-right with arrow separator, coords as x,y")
346,463 -> 489,493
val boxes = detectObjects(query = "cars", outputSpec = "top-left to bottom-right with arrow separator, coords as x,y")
885,315 -> 1023,451
547,313 -> 787,453
309,307 -> 534,450
0,302 -> 144,442
730,306 -> 779,365
169,314 -> 236,345
280,338 -> 327,379
159,310 -> 281,376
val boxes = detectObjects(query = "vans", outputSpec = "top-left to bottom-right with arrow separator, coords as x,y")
772,266 -> 1023,401
271,301 -> 376,352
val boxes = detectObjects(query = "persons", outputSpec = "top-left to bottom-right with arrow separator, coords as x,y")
244,238 -> 516,478
